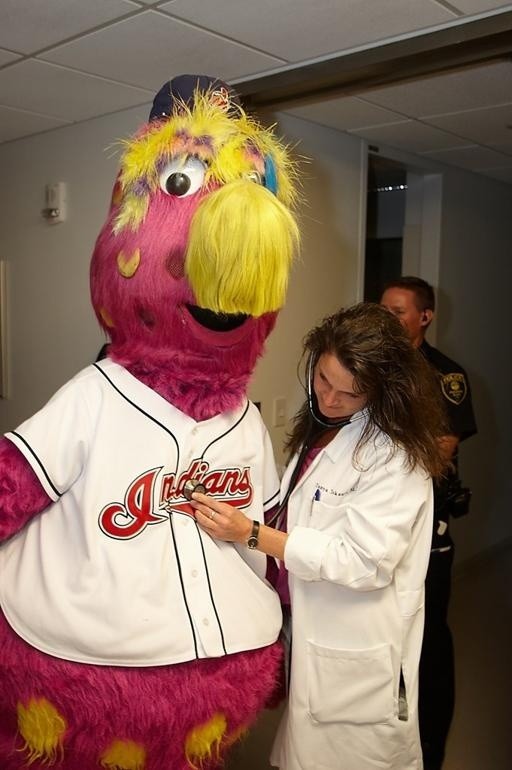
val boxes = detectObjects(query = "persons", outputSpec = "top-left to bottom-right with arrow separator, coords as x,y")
190,297 -> 460,770
378,275 -> 478,770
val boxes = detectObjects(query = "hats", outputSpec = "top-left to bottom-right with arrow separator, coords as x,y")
150,73 -> 257,122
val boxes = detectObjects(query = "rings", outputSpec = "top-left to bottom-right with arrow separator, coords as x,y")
209,512 -> 216,520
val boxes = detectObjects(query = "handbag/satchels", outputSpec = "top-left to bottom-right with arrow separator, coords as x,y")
446,480 -> 472,519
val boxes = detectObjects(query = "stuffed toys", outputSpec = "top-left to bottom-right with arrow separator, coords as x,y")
0,75 -> 286,770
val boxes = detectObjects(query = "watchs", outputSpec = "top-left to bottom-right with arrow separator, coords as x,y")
247,520 -> 259,550
